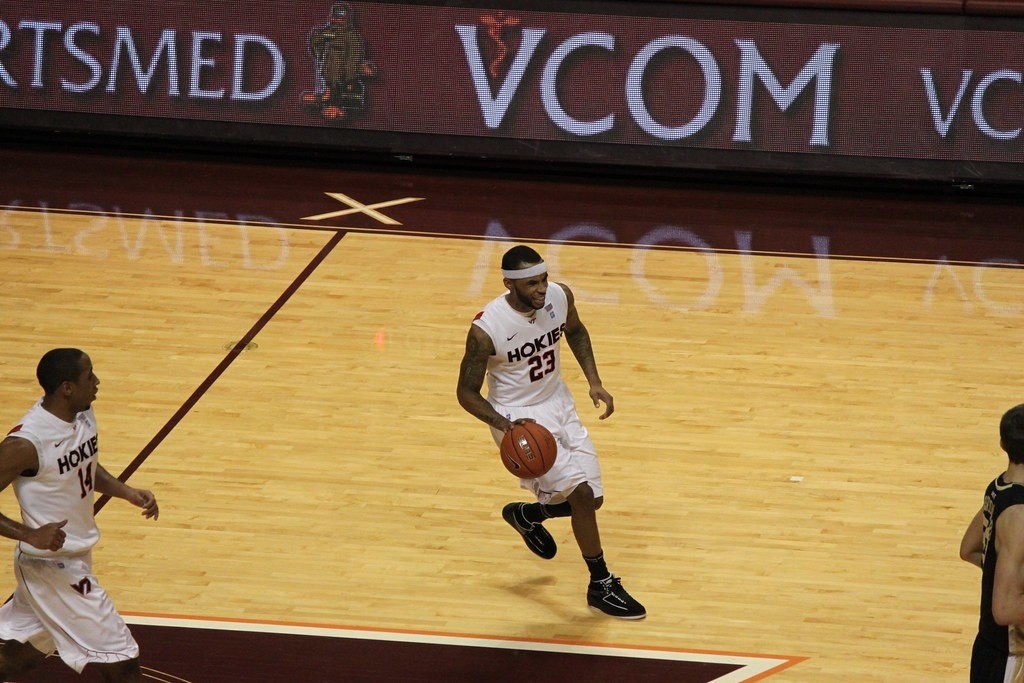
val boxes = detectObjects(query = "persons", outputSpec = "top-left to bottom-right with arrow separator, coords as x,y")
457,245 -> 648,620
0,349 -> 159,683
960,404 -> 1024,683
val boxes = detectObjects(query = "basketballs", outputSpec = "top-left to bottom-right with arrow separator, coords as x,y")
500,423 -> 558,480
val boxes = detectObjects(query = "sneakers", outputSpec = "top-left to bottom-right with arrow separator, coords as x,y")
501,501 -> 558,560
586,572 -> 647,620
